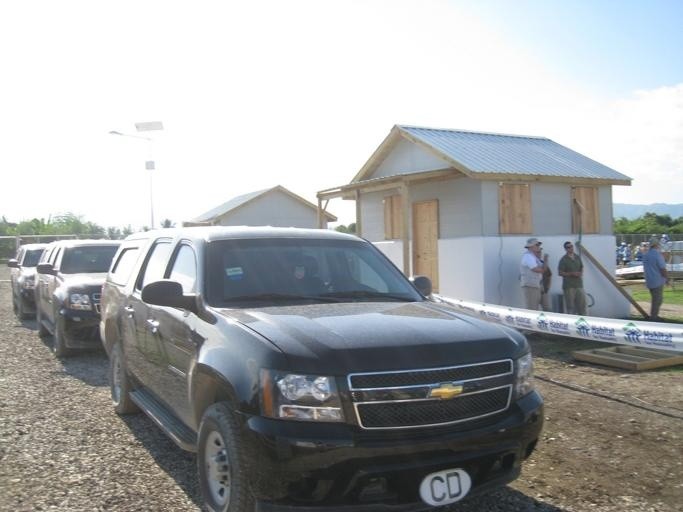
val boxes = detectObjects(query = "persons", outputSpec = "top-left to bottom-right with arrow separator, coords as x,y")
615,234 -> 673,285
518,236 -> 549,340
557,241 -> 589,315
533,247 -> 554,311
642,238 -> 675,320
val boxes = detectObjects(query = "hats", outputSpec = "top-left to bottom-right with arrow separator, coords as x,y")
525,238 -> 543,251
649,238 -> 658,246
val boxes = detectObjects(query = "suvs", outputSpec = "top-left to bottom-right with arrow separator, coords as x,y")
32,237 -> 125,358
4,243 -> 55,322
96,226 -> 547,512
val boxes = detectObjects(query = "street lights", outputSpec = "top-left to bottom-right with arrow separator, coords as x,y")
106,129 -> 157,228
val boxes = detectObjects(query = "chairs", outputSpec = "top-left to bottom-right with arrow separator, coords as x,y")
292,255 -> 330,297
